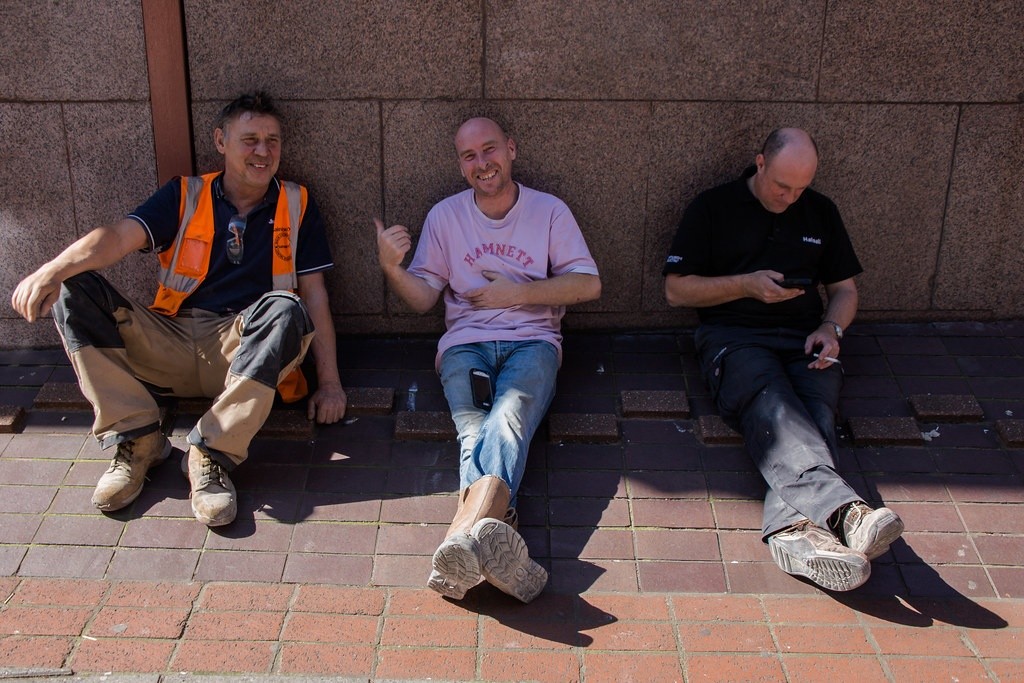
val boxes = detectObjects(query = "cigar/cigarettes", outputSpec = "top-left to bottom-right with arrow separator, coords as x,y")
813,353 -> 841,364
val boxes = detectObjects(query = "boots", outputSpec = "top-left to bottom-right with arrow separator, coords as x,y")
427,475 -> 512,600
469,507 -> 548,604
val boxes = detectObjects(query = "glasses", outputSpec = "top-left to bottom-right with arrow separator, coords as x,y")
225,212 -> 249,266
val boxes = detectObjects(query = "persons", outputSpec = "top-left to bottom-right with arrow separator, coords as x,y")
372,118 -> 601,605
12,89 -> 347,528
662,127 -> 904,591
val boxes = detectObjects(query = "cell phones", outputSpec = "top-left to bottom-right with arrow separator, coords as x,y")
470,367 -> 493,411
779,278 -> 813,288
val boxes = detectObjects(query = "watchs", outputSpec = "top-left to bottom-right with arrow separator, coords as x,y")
823,321 -> 843,339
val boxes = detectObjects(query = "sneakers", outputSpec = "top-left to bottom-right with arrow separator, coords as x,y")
830,501 -> 904,561
91,428 -> 171,511
768,518 -> 872,592
181,442 -> 237,526
